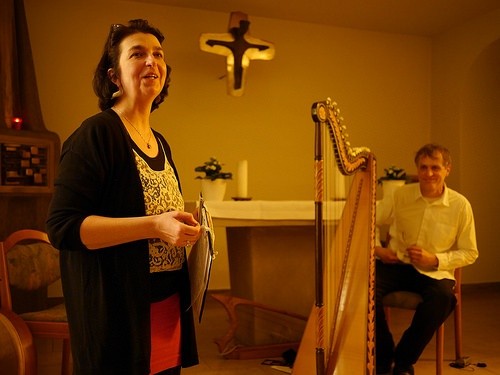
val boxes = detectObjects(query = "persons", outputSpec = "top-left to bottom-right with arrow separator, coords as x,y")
46,19 -> 202,375
375,143 -> 479,375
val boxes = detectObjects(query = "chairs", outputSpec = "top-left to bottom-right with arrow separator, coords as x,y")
0,229 -> 73,375
384,267 -> 463,375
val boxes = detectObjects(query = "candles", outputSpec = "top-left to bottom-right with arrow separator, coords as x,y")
237,160 -> 248,197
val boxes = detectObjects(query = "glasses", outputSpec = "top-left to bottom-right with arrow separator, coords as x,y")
110,23 -> 123,37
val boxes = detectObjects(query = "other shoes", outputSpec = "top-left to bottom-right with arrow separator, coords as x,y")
377,362 -> 396,375
393,365 -> 414,375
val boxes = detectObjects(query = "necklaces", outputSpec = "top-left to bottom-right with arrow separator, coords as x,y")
113,105 -> 152,149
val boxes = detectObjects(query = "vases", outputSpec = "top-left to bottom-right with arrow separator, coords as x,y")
201,180 -> 226,201
383,180 -> 404,198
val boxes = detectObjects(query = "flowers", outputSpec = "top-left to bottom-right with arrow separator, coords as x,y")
378,165 -> 406,180
194,157 -> 233,180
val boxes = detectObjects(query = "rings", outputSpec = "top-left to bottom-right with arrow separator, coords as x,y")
186,240 -> 191,248
